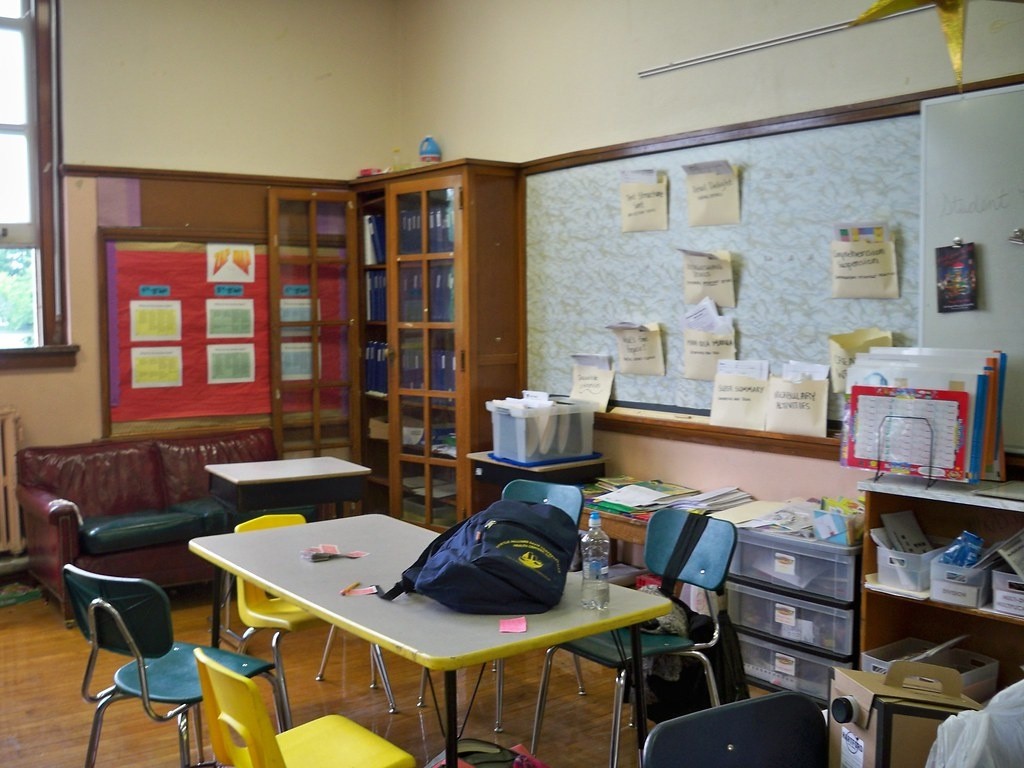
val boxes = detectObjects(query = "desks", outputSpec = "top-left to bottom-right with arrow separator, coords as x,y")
467,446 -> 610,557
579,474 -> 651,568
204,456 -> 372,648
188,514 -> 671,768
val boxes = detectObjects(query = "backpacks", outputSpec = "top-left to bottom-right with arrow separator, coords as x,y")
369,499 -> 579,615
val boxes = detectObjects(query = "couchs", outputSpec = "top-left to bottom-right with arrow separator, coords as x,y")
16,426 -> 326,631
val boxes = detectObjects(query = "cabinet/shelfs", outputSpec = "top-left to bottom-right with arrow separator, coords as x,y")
267,158 -> 525,534
860,478 -> 1023,677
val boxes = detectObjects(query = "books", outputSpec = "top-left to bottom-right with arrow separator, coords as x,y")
578,475 -> 789,529
492,398 -> 583,458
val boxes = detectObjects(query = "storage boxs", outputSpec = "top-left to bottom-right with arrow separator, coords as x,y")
990,565 -> 1024,616
876,546 -> 946,592
827,661 -> 985,768
930,550 -> 992,608
724,531 -> 863,606
486,396 -> 597,467
735,633 -> 859,701
861,638 -> 998,703
724,580 -> 860,658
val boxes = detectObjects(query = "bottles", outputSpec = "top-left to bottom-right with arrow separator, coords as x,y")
581,513 -> 609,611
392,149 -> 400,172
418,136 -> 441,162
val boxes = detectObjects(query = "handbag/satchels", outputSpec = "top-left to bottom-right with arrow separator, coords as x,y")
631,513 -> 753,722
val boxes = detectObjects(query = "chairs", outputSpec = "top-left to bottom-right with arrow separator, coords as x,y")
420,479 -> 583,732
532,508 -> 738,768
642,691 -> 830,768
191,646 -> 415,768
61,561 -> 287,768
234,513 -> 401,730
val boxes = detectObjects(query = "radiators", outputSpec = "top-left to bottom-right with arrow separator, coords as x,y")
0,407 -> 29,558
